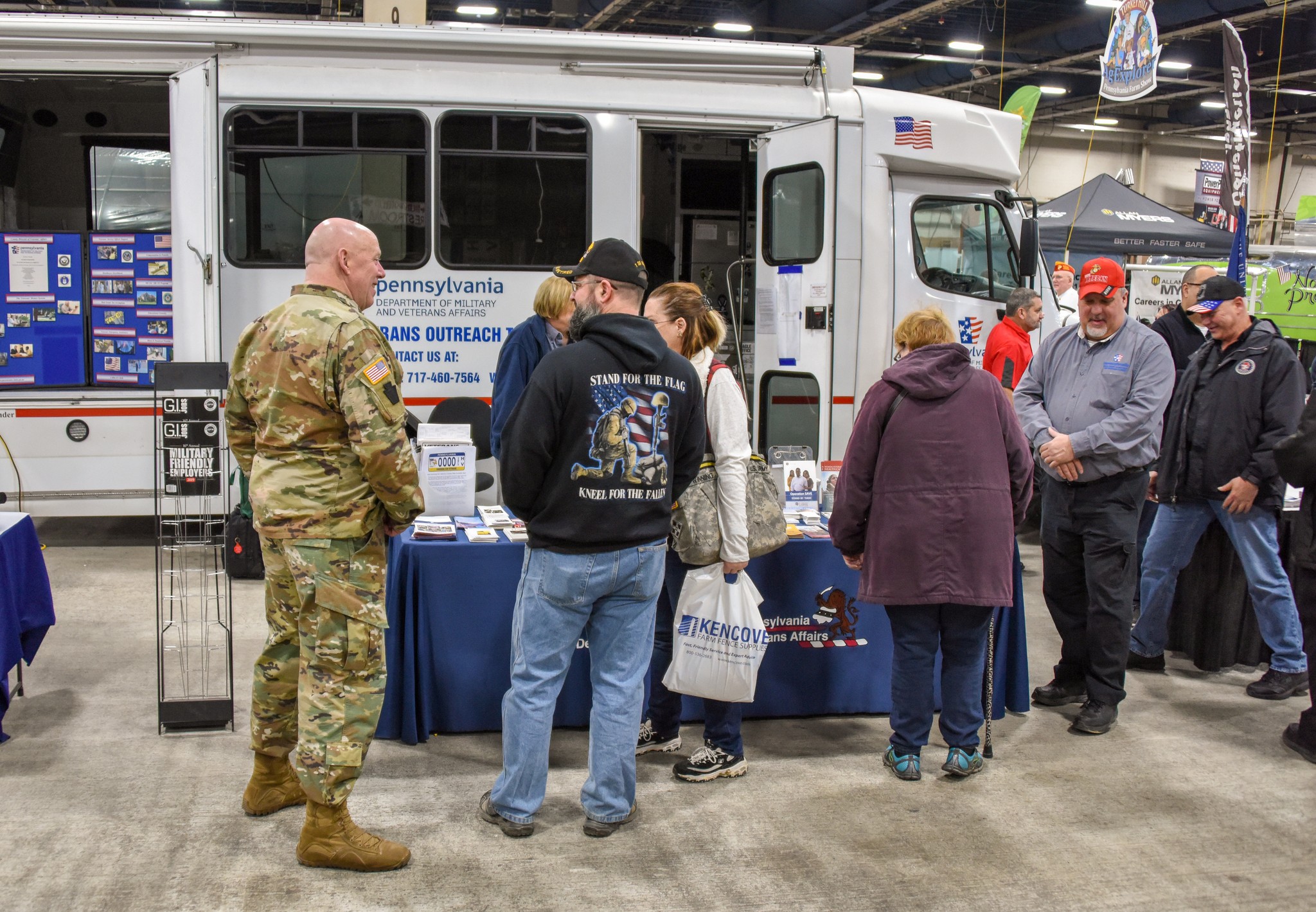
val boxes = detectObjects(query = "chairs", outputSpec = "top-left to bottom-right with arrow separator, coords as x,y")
426,396 -> 495,494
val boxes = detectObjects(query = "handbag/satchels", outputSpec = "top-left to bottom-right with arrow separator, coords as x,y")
662,452 -> 790,566
663,562 -> 767,707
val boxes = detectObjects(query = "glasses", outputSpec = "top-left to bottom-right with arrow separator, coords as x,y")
891,347 -> 910,365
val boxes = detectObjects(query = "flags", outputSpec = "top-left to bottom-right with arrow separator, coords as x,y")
1219,19 -> 1250,299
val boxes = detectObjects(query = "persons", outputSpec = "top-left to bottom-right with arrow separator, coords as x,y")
1155,304 -> 1183,326
1010,254 -> 1179,737
802,469 -> 814,492
222,217 -> 427,872
827,307 -> 1034,780
1117,272 -> 1309,702
488,273 -> 582,470
618,277 -> 755,782
826,474 -> 840,494
790,468 -> 808,492
787,470 -> 795,491
1147,263 -> 1220,474
1046,261 -> 1082,328
979,285 -> 1047,571
477,230 -> 708,840
1272,328 -> 1315,760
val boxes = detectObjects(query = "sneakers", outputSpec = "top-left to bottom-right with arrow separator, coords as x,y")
585,796 -> 642,840
882,743 -> 922,782
296,799 -> 417,875
1032,677 -> 1084,706
477,784 -> 538,839
1281,720 -> 1316,765
1074,699 -> 1120,738
940,745 -> 987,779
1246,667 -> 1310,701
242,746 -> 311,818
631,718 -> 682,759
1125,649 -> 1168,670
672,737 -> 751,785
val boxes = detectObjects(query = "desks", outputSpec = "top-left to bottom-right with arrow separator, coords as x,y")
0,511 -> 56,745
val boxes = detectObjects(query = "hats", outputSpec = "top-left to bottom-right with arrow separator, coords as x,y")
1186,275 -> 1247,317
1077,257 -> 1128,300
1052,261 -> 1075,276
553,238 -> 651,297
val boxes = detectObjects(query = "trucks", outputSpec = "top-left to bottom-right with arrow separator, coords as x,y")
0,9 -> 1075,524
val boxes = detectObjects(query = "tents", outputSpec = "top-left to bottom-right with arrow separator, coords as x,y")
1025,169 -> 1239,315
